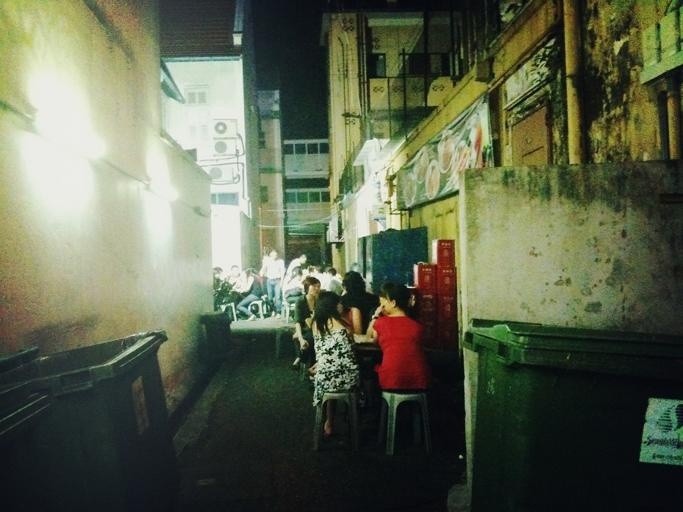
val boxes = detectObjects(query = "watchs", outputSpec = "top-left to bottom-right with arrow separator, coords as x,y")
370,314 -> 379,320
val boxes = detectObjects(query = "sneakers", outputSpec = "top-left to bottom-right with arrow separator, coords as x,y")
323,422 -> 333,437
247,314 -> 256,321
289,361 -> 316,382
272,311 -> 280,319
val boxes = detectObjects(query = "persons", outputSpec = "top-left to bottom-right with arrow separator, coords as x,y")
365,281 -> 432,443
333,269 -> 381,334
213,244 -> 344,326
333,294 -> 363,408
309,290 -> 364,441
292,275 -> 319,389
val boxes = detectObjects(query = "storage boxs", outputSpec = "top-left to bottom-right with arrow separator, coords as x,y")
413,238 -> 458,349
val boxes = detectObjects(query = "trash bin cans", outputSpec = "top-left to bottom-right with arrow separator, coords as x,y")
200,312 -> 231,353
0,328 -> 168,512
463,319 -> 683,512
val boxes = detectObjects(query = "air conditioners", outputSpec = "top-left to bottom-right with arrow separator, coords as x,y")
205,118 -> 237,183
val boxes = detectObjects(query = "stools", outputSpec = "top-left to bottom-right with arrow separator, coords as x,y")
216,300 -> 295,323
312,389 -> 431,456
